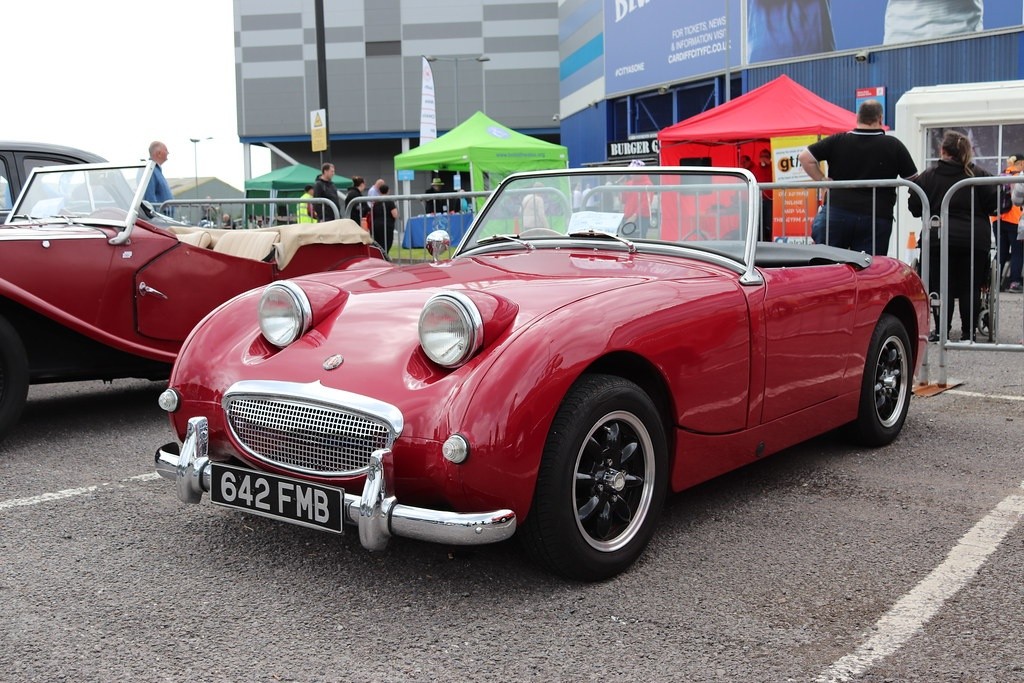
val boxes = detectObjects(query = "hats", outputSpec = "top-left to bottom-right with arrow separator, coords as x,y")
430,178 -> 444,185
627,160 -> 645,166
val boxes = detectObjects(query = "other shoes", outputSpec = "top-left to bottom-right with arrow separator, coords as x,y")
959,334 -> 976,343
928,330 -> 951,343
1009,282 -> 1023,293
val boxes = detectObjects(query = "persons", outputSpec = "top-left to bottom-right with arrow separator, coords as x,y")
1008,170 -> 1024,293
799,99 -> 919,258
198,164 -> 614,252
739,155 -> 755,172
622,159 -> 655,239
751,149 -> 773,242
907,130 -> 1012,339
987,153 -> 1024,279
135,140 -> 175,220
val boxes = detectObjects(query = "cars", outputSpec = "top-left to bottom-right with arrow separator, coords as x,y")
0,140 -> 390,441
153,167 -> 930,581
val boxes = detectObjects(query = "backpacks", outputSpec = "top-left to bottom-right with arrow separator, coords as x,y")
989,169 -> 1021,216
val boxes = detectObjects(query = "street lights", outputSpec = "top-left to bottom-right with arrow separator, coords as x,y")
426,52 -> 491,190
190,138 -> 201,199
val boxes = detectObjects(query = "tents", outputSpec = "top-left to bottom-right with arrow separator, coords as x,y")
242,163 -> 353,230
382,111 -> 572,236
658,74 -> 890,242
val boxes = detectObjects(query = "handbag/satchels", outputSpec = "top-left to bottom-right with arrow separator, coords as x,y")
367,201 -> 376,231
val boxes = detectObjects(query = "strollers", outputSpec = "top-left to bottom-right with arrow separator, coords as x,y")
977,244 -> 1011,337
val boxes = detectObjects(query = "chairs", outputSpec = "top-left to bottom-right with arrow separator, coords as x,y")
174,231 -> 211,248
212,230 -> 280,262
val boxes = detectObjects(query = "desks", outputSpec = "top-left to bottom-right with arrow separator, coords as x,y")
402,212 -> 472,249
